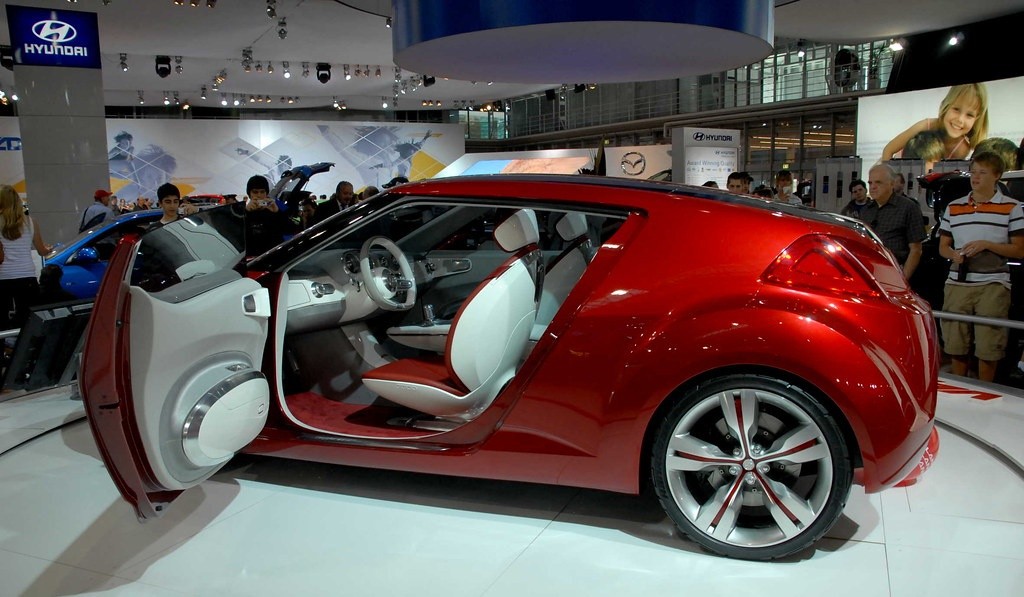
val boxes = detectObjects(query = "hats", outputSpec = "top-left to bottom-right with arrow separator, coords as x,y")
94,190 -> 113,199
381,176 -> 409,189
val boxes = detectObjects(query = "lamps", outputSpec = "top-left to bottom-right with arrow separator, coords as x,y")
950,32 -> 964,46
221,93 -> 228,106
213,76 -> 218,90
182,100 -> 189,110
216,70 -> 228,84
154,55 -> 172,78
162,91 -> 171,105
233,0 -> 511,112
173,92 -> 180,105
890,37 -> 908,52
119,53 -> 129,72
200,85 -> 207,100
0,86 -> 18,105
544,88 -> 557,101
574,83 -> 598,94
175,56 -> 183,74
138,90 -> 145,104
559,84 -> 568,100
797,42 -> 806,57
174,0 -> 217,8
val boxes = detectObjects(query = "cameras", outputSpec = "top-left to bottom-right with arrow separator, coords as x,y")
258,199 -> 268,206
178,207 -> 185,215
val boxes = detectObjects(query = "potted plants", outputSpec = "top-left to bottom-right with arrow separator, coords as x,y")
867,40 -> 890,89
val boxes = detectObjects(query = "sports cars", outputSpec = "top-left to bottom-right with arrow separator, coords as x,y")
81,172 -> 940,565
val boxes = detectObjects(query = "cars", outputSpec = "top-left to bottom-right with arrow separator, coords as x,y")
188,193 -> 225,205
45,203 -> 209,303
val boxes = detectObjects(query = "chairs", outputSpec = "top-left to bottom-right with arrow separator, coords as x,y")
537,213 -> 596,326
361,209 -> 545,418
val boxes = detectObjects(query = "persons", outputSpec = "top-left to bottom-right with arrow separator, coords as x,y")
883,83 -> 1024,171
0,185 -> 77,372
78,175 -> 409,257
108,133 -> 178,193
235,147 -> 292,186
702,164 -> 927,282
320,126 -> 433,190
938,152 -> 1024,382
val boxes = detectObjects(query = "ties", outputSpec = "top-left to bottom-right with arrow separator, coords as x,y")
340,204 -> 346,211
